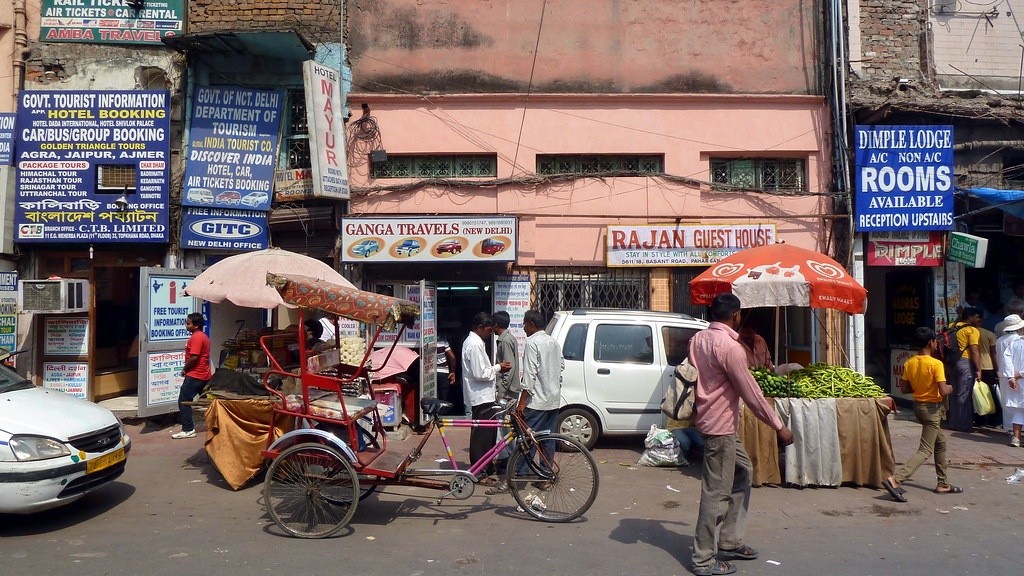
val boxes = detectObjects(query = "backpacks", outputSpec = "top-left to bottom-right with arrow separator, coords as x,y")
935,321 -> 973,363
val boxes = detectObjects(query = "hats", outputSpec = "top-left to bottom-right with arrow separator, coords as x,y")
1002,314 -> 1024,331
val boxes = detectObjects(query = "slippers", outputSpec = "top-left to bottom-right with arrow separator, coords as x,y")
936,484 -> 964,493
485,480 -> 509,494
534,475 -> 559,490
881,480 -> 908,502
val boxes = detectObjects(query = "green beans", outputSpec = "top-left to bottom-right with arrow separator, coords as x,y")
784,362 -> 887,402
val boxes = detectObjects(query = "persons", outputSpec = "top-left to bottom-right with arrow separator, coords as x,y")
947,296 -> 1024,448
437,331 -> 456,416
689,291 -> 794,575
736,318 -> 774,369
171,312 -> 211,439
305,313 -> 337,345
461,310 -> 565,495
880,327 -> 965,501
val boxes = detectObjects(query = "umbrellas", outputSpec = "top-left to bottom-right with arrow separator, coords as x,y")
183,245 -> 359,330
689,238 -> 868,367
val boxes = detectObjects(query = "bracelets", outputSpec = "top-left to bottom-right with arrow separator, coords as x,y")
1007,377 -> 1015,382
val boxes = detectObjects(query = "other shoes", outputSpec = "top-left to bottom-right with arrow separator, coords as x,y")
695,560 -> 736,575
717,545 -> 760,559
476,473 -> 498,486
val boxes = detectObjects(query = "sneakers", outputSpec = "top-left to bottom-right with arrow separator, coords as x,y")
172,426 -> 196,438
1009,436 -> 1024,446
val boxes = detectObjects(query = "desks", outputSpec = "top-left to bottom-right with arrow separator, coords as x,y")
372,381 -> 402,432
739,396 -> 898,488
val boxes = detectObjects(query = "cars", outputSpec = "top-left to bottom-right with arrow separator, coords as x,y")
543,308 -> 714,453
0,361 -> 133,513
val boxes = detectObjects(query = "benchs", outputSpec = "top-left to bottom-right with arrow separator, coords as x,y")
260,332 -> 386,467
94,340 -> 138,403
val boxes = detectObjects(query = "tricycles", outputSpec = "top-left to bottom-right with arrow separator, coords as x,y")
261,270 -> 601,541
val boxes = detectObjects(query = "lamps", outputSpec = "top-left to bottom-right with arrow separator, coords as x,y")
116,182 -> 129,212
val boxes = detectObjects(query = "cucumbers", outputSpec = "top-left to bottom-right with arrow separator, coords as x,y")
748,365 -> 799,398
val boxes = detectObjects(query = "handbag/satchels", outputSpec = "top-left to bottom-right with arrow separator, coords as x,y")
972,377 -> 995,416
659,356 -> 698,420
493,409 -> 518,460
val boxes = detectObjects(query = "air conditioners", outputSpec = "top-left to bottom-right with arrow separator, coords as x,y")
17,279 -> 88,313
